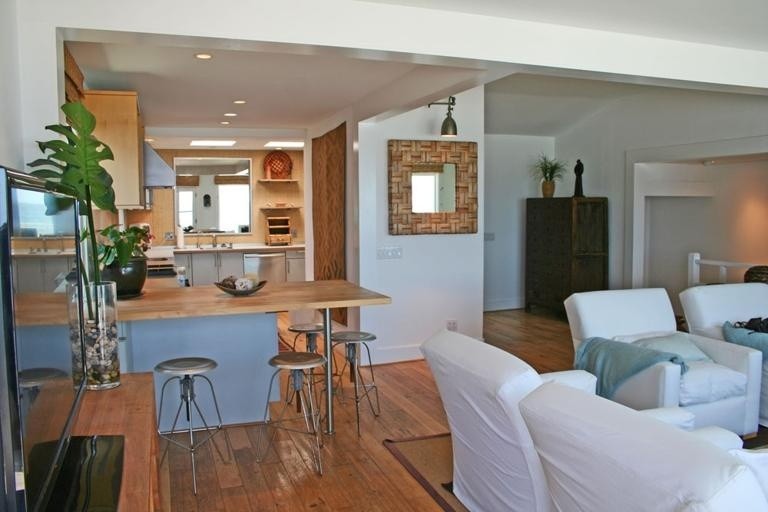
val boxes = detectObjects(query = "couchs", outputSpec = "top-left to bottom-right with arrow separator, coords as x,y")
518,379 -> 768,512
563,287 -> 763,440
678,282 -> 768,428
419,328 -> 696,512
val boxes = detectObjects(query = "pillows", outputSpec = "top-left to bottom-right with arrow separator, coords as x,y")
726,449 -> 768,502
722,321 -> 768,360
679,360 -> 748,406
630,333 -> 715,362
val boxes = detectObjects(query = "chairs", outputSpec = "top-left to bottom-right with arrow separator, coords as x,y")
319,331 -> 380,436
154,357 -> 232,495
256,352 -> 328,476
17,368 -> 68,397
285,323 -> 344,410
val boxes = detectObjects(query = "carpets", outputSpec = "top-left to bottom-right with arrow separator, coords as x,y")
382,432 -> 470,511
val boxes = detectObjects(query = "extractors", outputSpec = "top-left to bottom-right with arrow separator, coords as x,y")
144,142 -> 177,189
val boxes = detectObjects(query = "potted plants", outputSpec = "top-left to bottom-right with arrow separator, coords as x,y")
44,194 -> 84,390
26,99 -> 149,389
528,151 -> 569,197
79,223 -> 156,300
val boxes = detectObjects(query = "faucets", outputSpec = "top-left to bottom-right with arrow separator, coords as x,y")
40,237 -> 48,252
211,233 -> 218,247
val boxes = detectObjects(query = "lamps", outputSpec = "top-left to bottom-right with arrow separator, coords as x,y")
427,96 -> 457,137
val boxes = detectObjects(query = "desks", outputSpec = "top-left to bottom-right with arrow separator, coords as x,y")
118,279 -> 392,435
15,292 -> 73,380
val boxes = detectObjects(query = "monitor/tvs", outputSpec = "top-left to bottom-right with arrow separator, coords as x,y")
0,165 -> 125,512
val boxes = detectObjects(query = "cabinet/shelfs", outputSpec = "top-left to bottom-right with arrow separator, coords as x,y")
173,244 -> 192,287
67,248 -> 77,271
70,371 -> 162,512
525,197 -> 609,320
85,91 -> 146,210
265,217 -> 292,245
258,179 -> 303,210
17,248 -> 67,292
192,244 -> 243,285
11,248 -> 19,292
285,243 -> 305,283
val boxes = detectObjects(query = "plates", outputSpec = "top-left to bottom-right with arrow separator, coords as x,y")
213,279 -> 267,297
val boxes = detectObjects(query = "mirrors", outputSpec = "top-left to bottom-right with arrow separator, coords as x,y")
173,157 -> 253,237
410,161 -> 456,214
